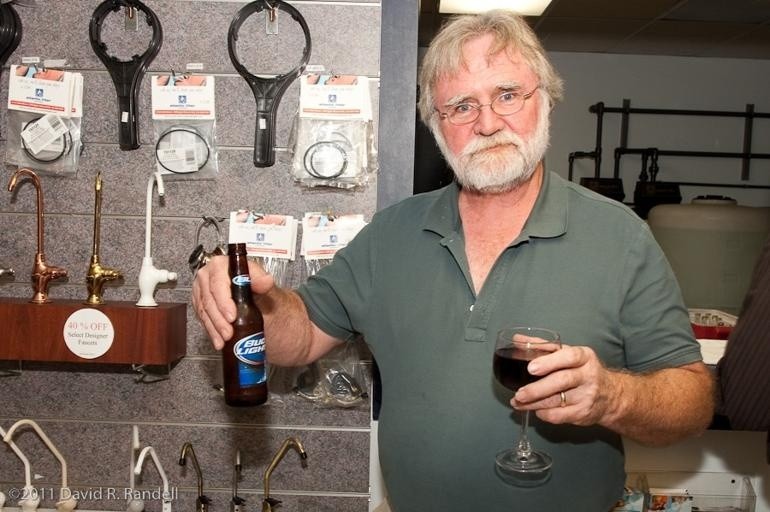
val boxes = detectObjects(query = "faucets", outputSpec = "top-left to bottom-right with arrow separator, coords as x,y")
180,442 -> 211,512
5,419 -> 78,510
135,447 -> 174,512
137,171 -> 178,308
262,437 -> 307,511
232,447 -> 246,512
7,166 -> 69,303
128,426 -> 145,510
84,170 -> 123,303
0,428 -> 41,510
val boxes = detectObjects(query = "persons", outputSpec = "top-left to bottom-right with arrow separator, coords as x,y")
191,7 -> 714,510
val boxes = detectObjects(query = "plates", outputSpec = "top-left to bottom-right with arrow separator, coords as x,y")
696,339 -> 728,365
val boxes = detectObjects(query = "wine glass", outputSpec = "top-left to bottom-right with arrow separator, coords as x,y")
494,328 -> 564,474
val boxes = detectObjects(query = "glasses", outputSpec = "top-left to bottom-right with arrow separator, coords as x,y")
435,87 -> 538,126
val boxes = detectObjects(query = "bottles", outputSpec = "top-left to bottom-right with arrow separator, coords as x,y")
222,242 -> 266,407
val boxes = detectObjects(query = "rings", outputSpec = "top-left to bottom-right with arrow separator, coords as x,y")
559,392 -> 569,409
